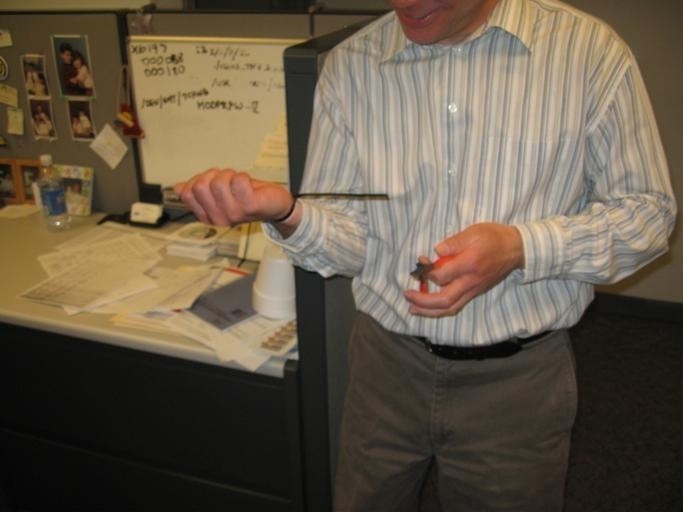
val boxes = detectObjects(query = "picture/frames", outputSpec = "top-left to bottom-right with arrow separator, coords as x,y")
14,158 -> 45,206
0,156 -> 17,206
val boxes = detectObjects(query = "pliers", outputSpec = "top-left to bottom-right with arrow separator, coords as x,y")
409,255 -> 465,293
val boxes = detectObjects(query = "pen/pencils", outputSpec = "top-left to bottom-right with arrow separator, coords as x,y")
213,262 -> 250,278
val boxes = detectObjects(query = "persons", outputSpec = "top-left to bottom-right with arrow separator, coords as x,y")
168,1 -> 678,512
21,42 -> 95,139
65,176 -> 83,197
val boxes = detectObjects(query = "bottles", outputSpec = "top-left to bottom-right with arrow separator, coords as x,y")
33,150 -> 70,234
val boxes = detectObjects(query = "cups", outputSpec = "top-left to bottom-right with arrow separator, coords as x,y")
249,247 -> 296,319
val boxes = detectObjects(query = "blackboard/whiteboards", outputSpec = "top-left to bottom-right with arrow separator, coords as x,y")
126,36 -> 308,185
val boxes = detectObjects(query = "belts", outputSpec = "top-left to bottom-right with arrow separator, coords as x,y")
415,331 -> 550,360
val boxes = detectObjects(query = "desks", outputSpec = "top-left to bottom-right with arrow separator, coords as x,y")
0,6 -> 390,512
